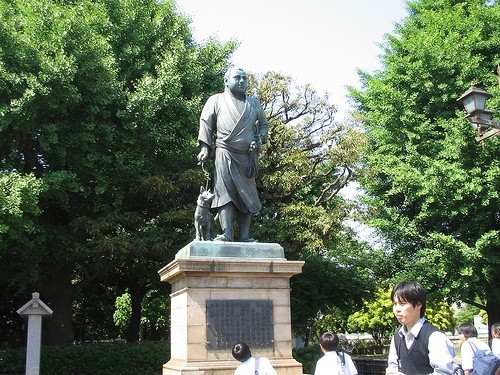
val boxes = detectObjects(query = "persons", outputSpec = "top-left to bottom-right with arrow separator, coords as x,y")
314,332 -> 358,375
232,342 -> 278,375
458,323 -> 492,375
196,67 -> 268,242
491,322 -> 500,375
385,281 -> 456,375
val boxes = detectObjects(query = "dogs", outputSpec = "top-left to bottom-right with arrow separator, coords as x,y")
194,185 -> 214,242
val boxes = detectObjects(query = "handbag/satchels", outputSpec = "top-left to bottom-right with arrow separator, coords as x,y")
468,341 -> 499,375
